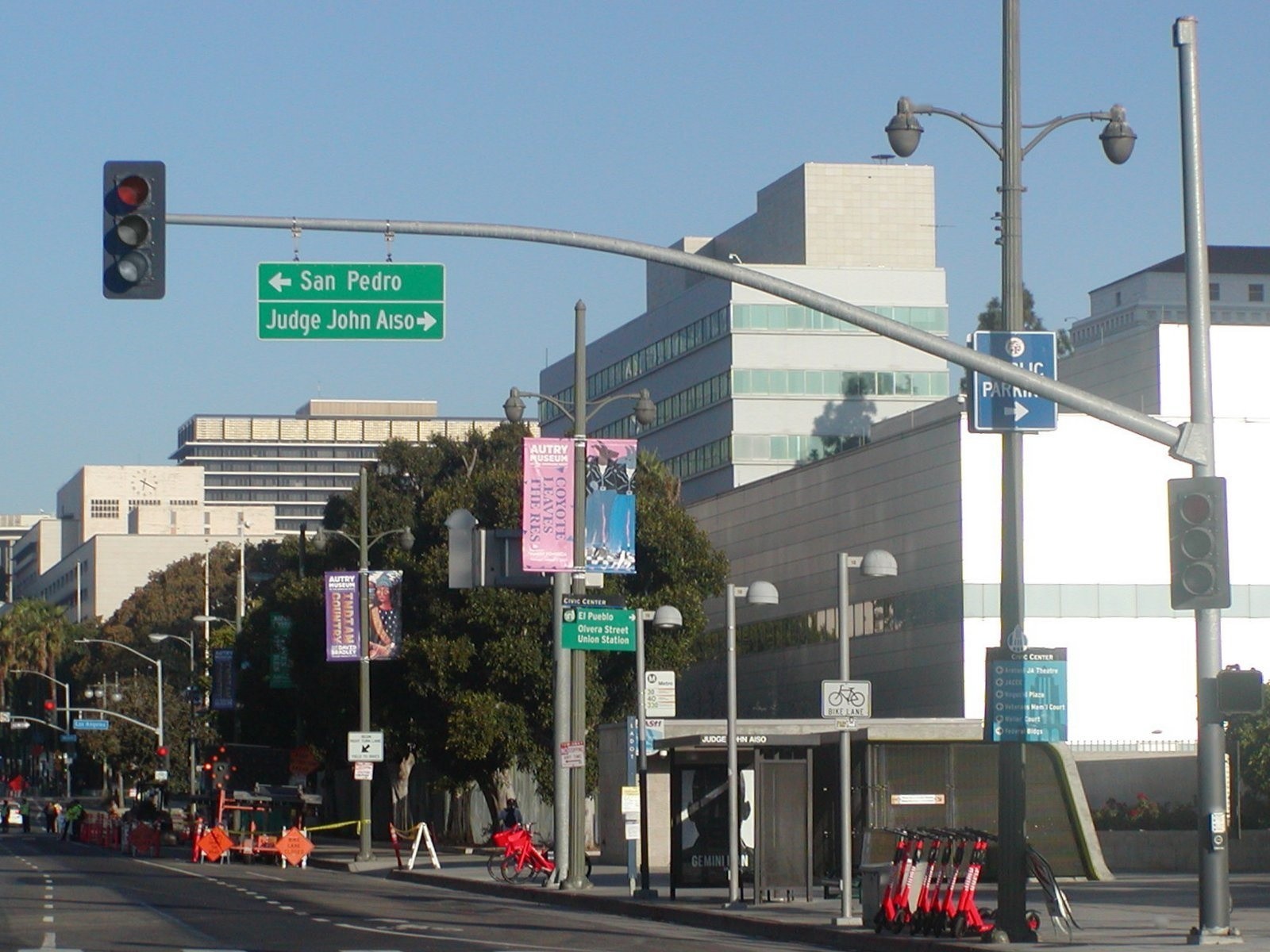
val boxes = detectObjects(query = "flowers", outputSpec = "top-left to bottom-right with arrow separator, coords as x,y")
1098,791 -> 1198,830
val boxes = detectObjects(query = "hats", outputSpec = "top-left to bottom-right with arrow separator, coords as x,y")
507,799 -> 519,809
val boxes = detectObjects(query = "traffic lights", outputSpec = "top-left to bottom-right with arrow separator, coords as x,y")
1215,664 -> 1264,716
156,745 -> 170,772
1165,475 -> 1233,612
99,158 -> 169,302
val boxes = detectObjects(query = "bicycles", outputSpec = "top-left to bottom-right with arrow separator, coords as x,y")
486,818 -> 592,886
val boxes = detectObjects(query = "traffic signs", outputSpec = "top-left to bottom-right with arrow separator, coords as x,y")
972,330 -> 1060,433
346,730 -> 387,764
255,261 -> 448,344
559,607 -> 637,653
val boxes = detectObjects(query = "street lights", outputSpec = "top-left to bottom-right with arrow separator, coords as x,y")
310,526 -> 418,867
8,669 -> 71,798
880,97 -> 1141,943
73,637 -> 165,747
630,605 -> 684,900
147,629 -> 197,819
831,548 -> 898,928
82,682 -> 123,709
720,580 -> 780,913
499,385 -> 657,889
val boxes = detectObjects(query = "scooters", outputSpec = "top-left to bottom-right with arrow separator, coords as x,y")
874,825 -> 1043,939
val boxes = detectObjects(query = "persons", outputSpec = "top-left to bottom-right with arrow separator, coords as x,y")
367,572 -> 401,658
1,799 -> 11,834
18,798 -> 32,833
43,799 -> 87,840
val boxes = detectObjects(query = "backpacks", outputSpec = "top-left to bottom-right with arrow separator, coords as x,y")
77,806 -> 86,819
503,808 -> 516,827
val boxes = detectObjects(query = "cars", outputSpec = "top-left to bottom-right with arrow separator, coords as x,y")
0,800 -> 23,827
